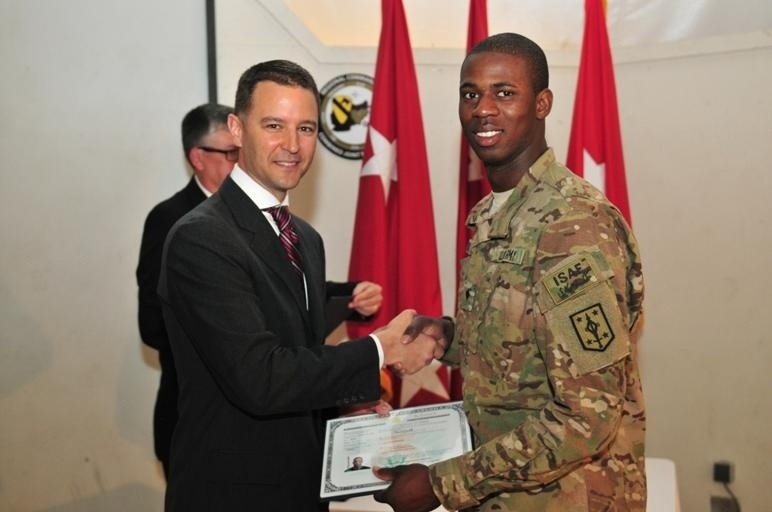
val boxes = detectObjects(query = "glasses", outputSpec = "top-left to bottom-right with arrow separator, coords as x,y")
200,147 -> 237,161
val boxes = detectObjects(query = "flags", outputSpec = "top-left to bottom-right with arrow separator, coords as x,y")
347,0 -> 451,411
453,0 -> 488,402
566,1 -> 631,224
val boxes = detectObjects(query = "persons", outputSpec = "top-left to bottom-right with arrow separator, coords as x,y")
157,61 -> 444,510
134,100 -> 384,483
373,32 -> 647,512
344,458 -> 370,474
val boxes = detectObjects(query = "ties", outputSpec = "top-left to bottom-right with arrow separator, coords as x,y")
268,205 -> 304,278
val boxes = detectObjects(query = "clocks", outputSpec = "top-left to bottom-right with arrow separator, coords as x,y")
316,74 -> 379,158
